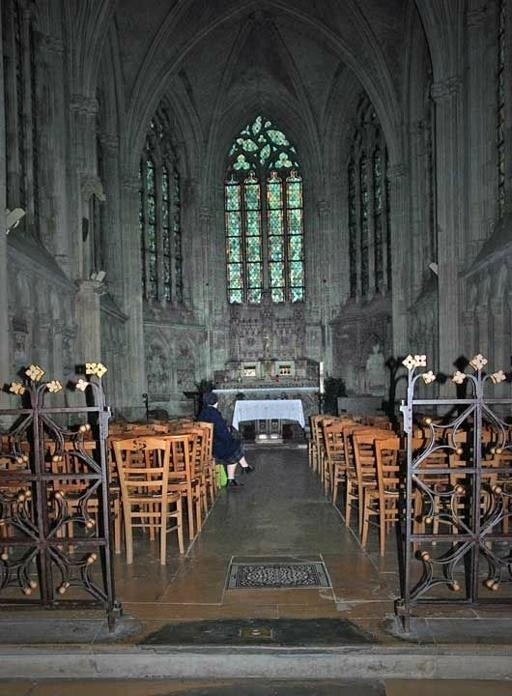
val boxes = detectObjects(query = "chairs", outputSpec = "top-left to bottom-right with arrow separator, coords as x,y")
0,416 -> 218,566
306,414 -> 511,558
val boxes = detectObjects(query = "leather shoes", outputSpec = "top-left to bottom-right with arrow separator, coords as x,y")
242,464 -> 255,474
227,479 -> 246,487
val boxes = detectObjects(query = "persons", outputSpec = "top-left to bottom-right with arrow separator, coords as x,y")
197,393 -> 254,489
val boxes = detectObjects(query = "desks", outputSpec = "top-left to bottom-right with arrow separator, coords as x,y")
232,398 -> 307,444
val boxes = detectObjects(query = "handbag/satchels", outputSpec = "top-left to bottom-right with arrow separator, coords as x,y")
215,464 -> 227,487
